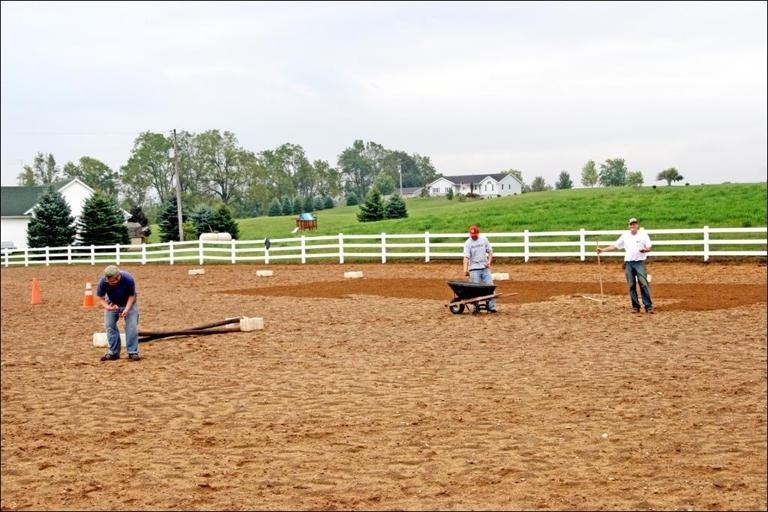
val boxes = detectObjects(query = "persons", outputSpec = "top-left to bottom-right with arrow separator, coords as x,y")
595,216 -> 653,313
463,224 -> 496,316
95,265 -> 141,362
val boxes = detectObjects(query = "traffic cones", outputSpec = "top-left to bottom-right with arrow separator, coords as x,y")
82,279 -> 99,310
29,276 -> 44,305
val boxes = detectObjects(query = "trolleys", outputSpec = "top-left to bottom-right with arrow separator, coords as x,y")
445,278 -> 518,315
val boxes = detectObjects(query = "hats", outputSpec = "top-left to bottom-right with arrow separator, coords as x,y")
469,225 -> 478,237
628,218 -> 637,224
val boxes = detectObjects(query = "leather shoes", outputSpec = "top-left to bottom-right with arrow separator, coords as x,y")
101,354 -> 120,361
128,353 -> 140,361
